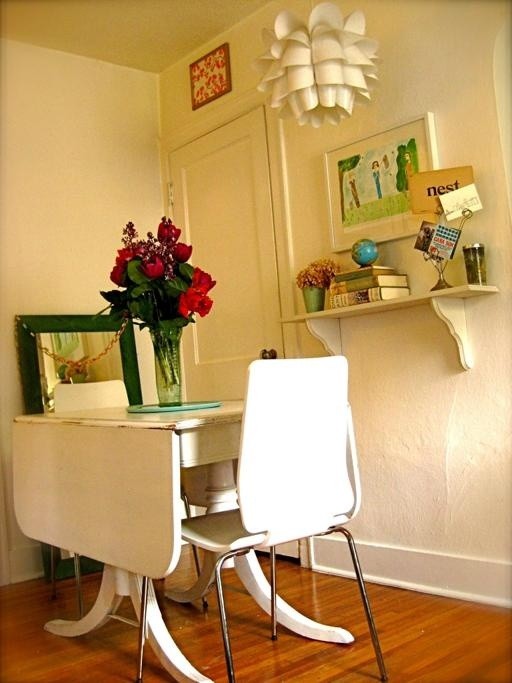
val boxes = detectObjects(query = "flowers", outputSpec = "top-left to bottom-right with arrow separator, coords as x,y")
292,257 -> 340,291
97,215 -> 220,384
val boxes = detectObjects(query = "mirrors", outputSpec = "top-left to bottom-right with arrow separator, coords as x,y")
16,312 -> 144,582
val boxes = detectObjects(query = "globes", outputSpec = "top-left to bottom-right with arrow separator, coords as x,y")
351,239 -> 379,269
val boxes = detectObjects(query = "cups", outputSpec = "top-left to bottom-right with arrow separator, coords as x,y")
462,243 -> 486,284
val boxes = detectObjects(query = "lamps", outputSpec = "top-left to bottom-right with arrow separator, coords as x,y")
253,0 -> 385,129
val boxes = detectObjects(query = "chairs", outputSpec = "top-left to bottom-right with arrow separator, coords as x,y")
137,356 -> 392,683
45,380 -> 210,619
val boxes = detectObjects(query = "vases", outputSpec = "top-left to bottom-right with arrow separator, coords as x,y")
143,319 -> 195,408
297,282 -> 328,311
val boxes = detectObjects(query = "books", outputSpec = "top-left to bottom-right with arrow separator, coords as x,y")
337,264 -> 394,273
330,286 -> 410,308
329,273 -> 408,296
335,269 -> 396,282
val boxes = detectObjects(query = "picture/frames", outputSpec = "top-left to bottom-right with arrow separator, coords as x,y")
50,332 -> 92,370
316,111 -> 450,256
184,44 -> 239,111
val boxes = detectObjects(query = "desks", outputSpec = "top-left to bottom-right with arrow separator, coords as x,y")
10,400 -> 355,683
